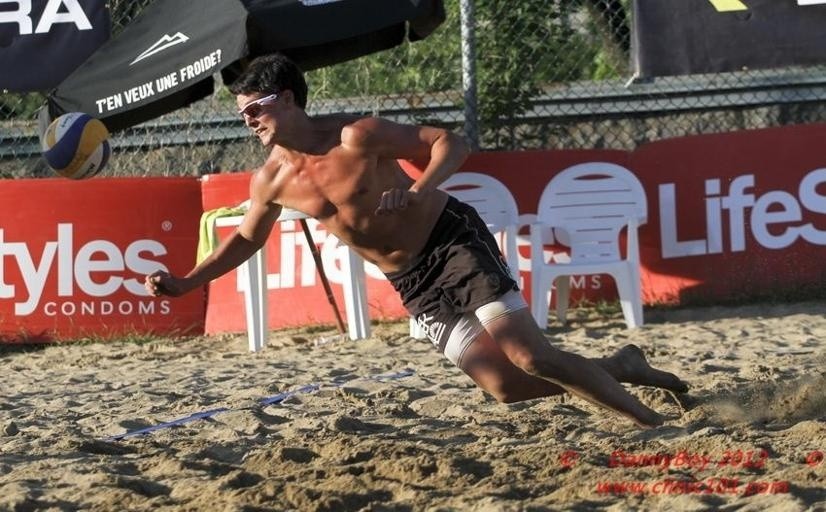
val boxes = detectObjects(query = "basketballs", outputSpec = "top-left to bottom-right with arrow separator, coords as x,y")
41,109 -> 114,180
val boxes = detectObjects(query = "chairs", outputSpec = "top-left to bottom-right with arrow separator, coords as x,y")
408,162 -> 650,340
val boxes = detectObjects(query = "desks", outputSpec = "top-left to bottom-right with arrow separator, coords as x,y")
209,207 -> 371,350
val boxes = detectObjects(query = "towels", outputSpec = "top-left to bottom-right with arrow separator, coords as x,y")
194,203 -> 248,270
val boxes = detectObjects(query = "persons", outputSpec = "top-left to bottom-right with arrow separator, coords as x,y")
145,54 -> 688,430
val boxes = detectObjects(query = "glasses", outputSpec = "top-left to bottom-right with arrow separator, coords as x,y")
238,94 -> 277,119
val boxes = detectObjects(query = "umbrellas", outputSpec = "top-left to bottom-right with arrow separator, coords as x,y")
37,0 -> 446,334
